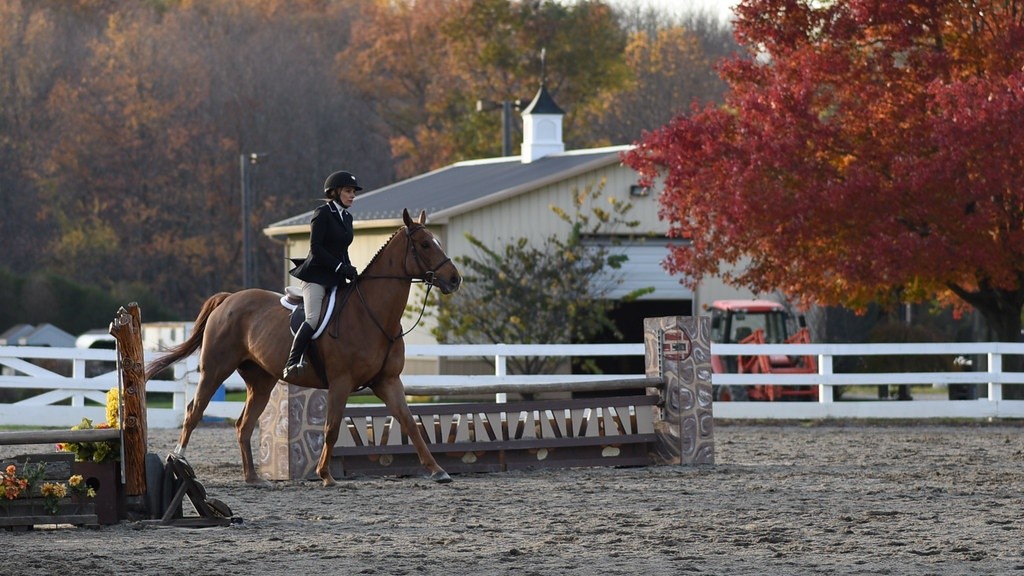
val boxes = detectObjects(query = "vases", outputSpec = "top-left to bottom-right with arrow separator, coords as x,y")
69,462 -> 121,525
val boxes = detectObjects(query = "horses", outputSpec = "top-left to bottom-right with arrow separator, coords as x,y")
144,206 -> 464,489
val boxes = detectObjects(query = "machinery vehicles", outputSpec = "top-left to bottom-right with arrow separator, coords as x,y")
701,300 -> 820,403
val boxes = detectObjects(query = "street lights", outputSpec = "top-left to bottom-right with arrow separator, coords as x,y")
475,98 -> 534,157
239,151 -> 269,289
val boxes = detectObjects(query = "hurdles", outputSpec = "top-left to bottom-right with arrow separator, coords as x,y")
0,295 -> 155,530
252,312 -> 716,483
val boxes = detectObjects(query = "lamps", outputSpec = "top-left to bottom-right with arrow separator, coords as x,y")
631,184 -> 650,197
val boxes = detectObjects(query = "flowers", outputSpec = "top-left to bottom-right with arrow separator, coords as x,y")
0,458 -> 96,519
54,386 -> 126,462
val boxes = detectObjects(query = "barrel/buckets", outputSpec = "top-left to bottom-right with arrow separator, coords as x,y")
201,385 -> 226,421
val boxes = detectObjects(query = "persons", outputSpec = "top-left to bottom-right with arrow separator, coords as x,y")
283,170 -> 363,381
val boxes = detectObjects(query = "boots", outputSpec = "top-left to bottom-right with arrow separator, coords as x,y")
283,322 -> 313,381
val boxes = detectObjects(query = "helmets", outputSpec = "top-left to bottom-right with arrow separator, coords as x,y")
324,171 -> 363,197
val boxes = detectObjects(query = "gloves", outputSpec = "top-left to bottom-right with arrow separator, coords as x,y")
337,263 -> 358,283
